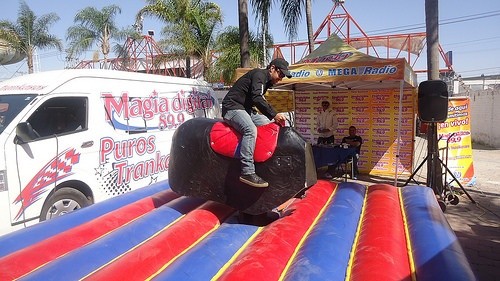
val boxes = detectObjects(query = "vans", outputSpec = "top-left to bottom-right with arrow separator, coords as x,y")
0,69 -> 221,238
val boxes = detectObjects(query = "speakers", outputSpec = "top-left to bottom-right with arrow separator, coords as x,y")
418,80 -> 448,123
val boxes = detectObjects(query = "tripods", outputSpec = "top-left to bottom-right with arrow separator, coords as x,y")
404,122 -> 476,205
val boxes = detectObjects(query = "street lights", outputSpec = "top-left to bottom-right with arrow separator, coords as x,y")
148,30 -> 154,74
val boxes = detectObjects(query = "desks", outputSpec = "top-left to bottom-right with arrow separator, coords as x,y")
312,146 -> 354,182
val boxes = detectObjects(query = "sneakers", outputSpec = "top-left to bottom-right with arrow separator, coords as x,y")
239,173 -> 269,187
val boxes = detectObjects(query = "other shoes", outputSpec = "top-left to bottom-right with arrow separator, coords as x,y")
332,177 -> 343,181
325,173 -> 332,179
353,175 -> 358,180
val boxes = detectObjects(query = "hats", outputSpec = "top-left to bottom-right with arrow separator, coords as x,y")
270,57 -> 292,78
319,98 -> 332,106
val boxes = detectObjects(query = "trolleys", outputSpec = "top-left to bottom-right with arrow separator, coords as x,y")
436,132 -> 459,212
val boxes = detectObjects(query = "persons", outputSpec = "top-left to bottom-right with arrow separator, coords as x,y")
316,97 -> 340,177
222,58 -> 292,187
326,126 -> 363,179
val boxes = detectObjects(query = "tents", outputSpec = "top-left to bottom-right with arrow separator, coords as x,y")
221,32 -> 417,187
